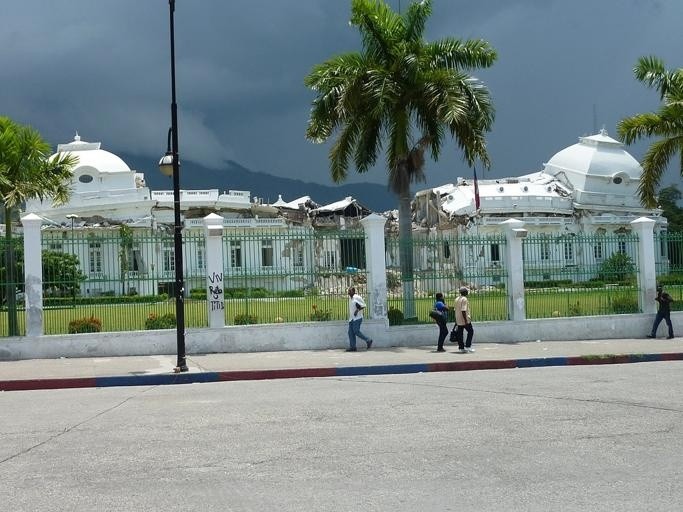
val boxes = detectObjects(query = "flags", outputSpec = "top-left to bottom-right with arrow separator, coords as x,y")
473,166 -> 479,210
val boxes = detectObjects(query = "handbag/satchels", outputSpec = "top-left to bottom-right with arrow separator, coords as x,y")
428,308 -> 446,323
449,323 -> 459,342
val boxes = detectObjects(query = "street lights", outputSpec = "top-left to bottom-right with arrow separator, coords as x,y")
158,126 -> 189,372
66,214 -> 78,307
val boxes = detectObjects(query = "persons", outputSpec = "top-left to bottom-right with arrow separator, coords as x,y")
433,291 -> 449,352
345,285 -> 372,352
453,286 -> 475,353
646,283 -> 675,339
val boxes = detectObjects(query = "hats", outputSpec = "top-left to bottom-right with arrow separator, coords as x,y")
458,287 -> 469,293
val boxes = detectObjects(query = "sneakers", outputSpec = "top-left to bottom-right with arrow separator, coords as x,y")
345,338 -> 373,352
437,346 -> 475,353
647,334 -> 674,339
